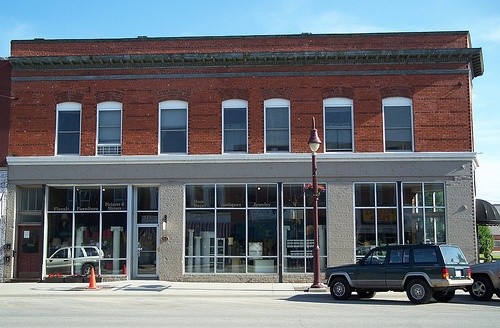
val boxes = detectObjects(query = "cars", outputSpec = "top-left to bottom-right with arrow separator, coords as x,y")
463,257 -> 500,301
326,240 -> 472,304
42,243 -> 106,277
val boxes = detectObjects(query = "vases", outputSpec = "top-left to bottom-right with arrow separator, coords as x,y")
83,277 -> 102,282
66,277 -> 83,283
47,278 -> 65,283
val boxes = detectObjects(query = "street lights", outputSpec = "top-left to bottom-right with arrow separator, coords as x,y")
305,116 -> 324,294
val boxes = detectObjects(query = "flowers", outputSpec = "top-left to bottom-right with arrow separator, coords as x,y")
84,273 -> 100,277
48,273 -> 63,278
68,274 -> 82,278
303,183 -> 325,195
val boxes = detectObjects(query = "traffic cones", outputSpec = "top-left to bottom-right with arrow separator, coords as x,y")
86,267 -> 98,291
